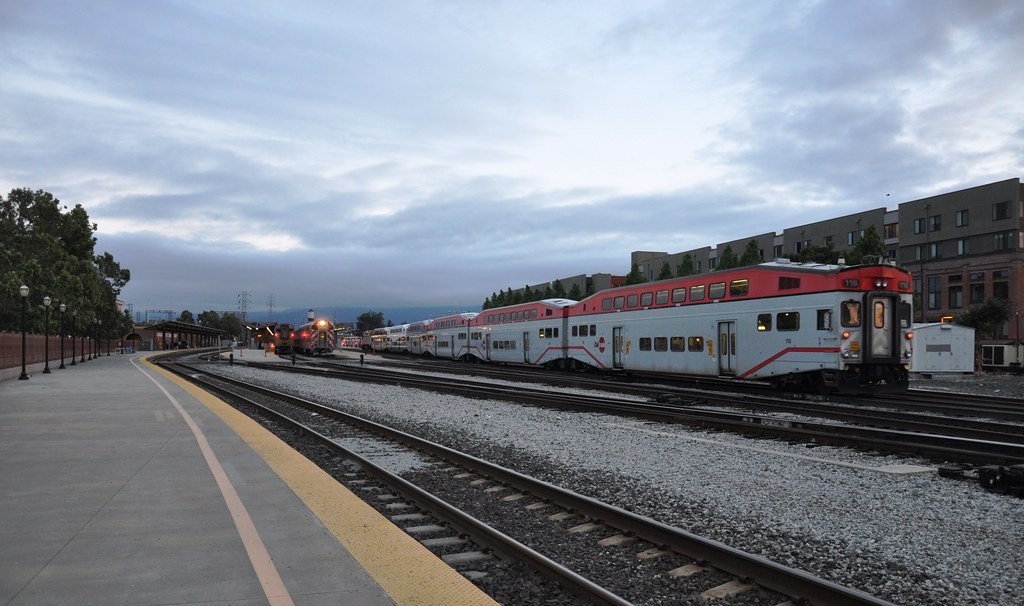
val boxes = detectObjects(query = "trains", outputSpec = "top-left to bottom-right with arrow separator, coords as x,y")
291,319 -> 339,357
359,257 -> 916,398
273,322 -> 296,355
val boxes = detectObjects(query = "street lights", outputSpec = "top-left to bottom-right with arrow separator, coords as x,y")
132,324 -> 136,353
43,296 -> 52,373
70,309 -> 77,365
93,318 -> 98,359
120,323 -> 124,354
97,320 -> 102,357
58,304 -> 66,369
18,284 -> 30,381
